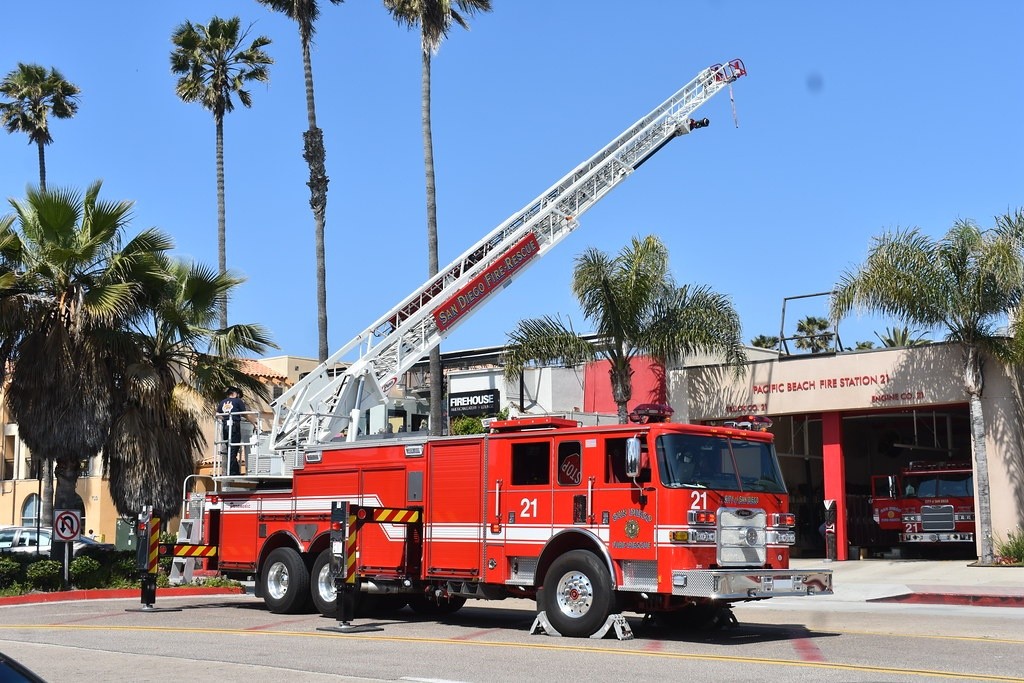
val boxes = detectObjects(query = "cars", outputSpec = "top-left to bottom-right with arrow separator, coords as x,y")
0,524 -> 99,559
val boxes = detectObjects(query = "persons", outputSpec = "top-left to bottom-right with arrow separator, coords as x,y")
88,529 -> 99,539
216,386 -> 257,475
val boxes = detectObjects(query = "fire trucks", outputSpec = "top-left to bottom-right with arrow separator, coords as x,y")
128,56 -> 832,643
868,458 -> 977,558
